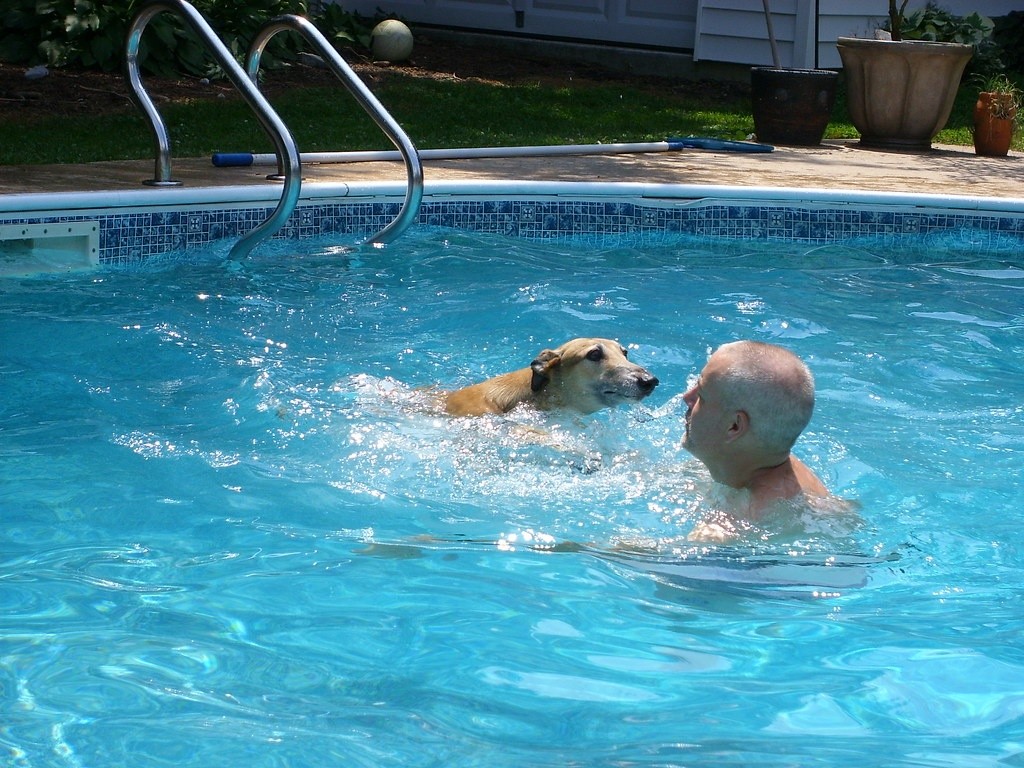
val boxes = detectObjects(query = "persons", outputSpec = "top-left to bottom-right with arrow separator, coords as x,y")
682,339 -> 833,522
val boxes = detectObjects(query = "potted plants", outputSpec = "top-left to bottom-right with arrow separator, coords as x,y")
837,0 -> 994,152
968,69 -> 1023,156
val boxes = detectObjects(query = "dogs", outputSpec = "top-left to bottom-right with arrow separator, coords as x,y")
447,336 -> 660,443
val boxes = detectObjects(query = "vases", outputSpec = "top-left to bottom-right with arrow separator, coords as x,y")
750,66 -> 838,145
